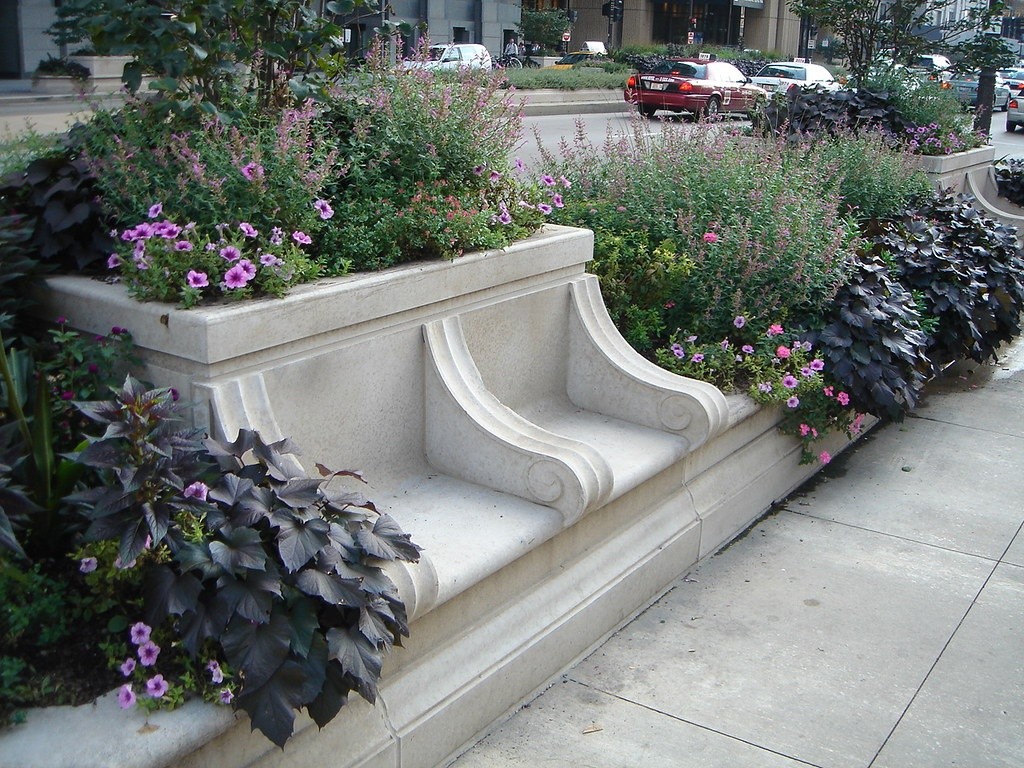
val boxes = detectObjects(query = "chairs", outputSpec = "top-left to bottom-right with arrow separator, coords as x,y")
935,165 -> 1024,240
193,274 -> 730,632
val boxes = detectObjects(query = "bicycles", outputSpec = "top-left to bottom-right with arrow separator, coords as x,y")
491,52 -> 541,70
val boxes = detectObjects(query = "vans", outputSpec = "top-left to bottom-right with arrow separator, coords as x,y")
388,43 -> 493,76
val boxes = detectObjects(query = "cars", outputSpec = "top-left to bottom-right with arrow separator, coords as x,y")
623,52 -> 769,125
936,68 -> 1012,113
745,56 -> 858,118
541,40 -> 640,76
1006,86 -> 1024,133
846,47 -> 1024,97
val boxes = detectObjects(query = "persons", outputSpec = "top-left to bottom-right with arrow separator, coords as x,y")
518,42 -> 527,56
531,41 -> 540,50
505,38 -> 518,56
